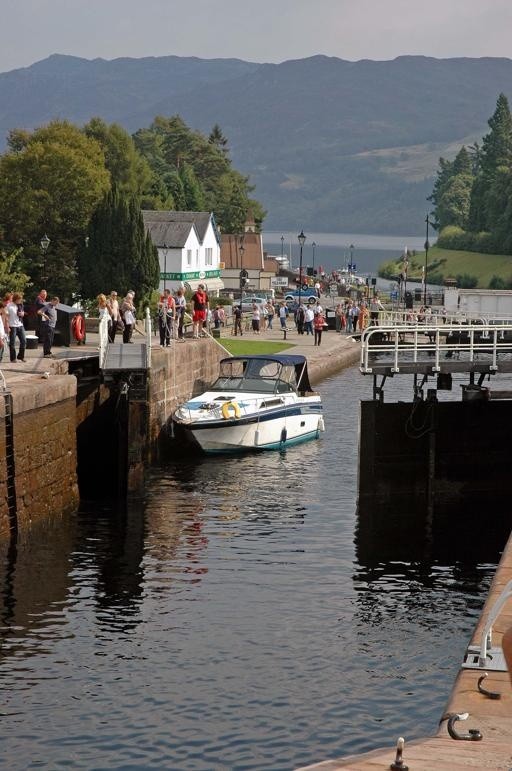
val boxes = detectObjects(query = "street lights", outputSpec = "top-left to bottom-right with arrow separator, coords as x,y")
281,234 -> 284,258
298,230 -> 306,290
40,232 -> 51,289
312,242 -> 316,276
161,243 -> 169,291
238,246 -> 245,292
350,244 -> 354,274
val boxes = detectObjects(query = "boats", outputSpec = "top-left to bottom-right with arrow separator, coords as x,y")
169,353 -> 323,458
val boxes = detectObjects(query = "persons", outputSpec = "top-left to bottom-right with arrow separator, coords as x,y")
190,284 -> 209,340
315,281 -> 320,299
371,295 -> 386,313
5,293 -> 27,364
440,308 -> 447,324
294,301 -> 369,335
277,302 -> 290,331
311,313 -> 328,347
125,290 -> 136,312
232,305 -> 243,337
252,304 -> 261,335
370,297 -> 380,327
157,289 -> 176,348
36,297 -> 60,359
32,290 -> 48,344
205,304 -> 226,332
266,301 -> 275,330
105,290 -> 125,344
405,305 -> 433,324
172,287 -> 188,343
120,293 -> 137,345
95,294 -> 114,343
0,292 -> 13,364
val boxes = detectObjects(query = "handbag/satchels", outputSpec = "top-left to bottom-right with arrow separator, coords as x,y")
124,309 -> 135,325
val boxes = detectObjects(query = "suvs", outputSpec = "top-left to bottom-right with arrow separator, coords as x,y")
285,286 -> 321,304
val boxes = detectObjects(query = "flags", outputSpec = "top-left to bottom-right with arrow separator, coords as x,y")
401,246 -> 409,283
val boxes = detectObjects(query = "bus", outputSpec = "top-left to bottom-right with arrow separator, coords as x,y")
235,289 -> 276,306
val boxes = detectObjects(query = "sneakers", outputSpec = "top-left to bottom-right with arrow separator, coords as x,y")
232,332 -> 243,336
298,331 -> 314,335
266,327 -> 273,331
178,337 -> 186,343
127,341 -> 133,344
253,330 -> 260,335
43,353 -> 56,359
160,344 -> 172,348
192,333 -> 204,339
11,358 -> 28,364
314,343 -> 320,346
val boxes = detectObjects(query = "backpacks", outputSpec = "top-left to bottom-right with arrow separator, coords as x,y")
120,306 -> 127,318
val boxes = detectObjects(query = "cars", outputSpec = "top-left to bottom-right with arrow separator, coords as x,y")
273,301 -> 300,314
232,296 -> 267,313
